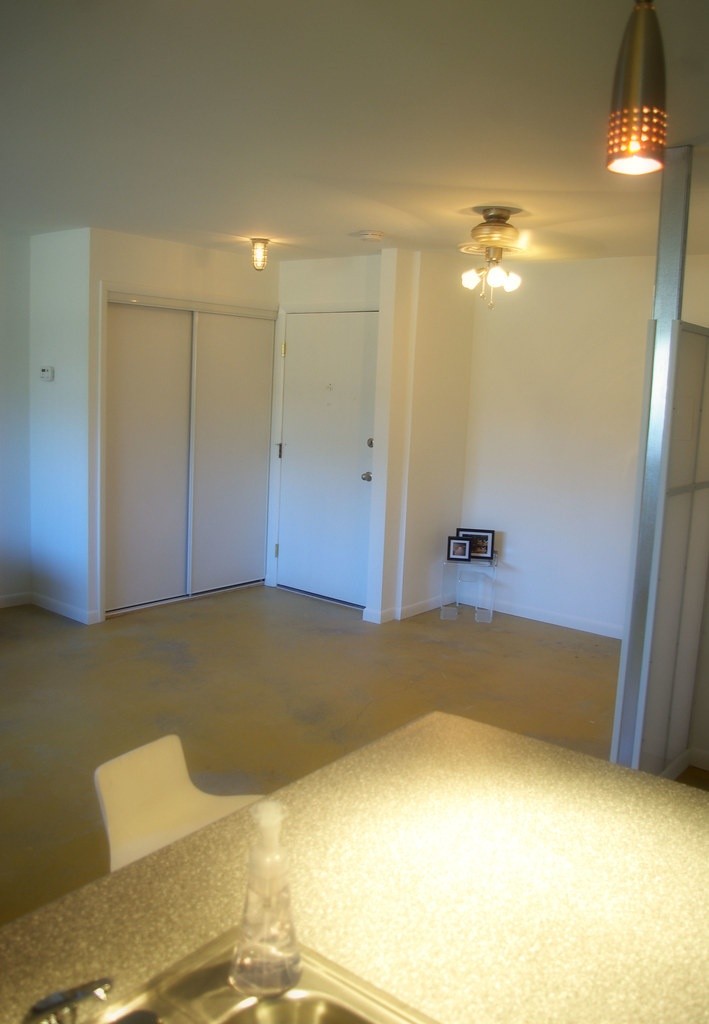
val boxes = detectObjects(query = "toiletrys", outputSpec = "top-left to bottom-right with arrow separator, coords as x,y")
226,801 -> 302,1000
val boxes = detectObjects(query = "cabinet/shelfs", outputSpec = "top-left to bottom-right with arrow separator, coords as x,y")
100,302 -> 275,614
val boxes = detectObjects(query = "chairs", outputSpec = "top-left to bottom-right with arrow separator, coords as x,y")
89,730 -> 265,873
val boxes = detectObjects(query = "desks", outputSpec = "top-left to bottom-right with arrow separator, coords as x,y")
440,559 -> 499,623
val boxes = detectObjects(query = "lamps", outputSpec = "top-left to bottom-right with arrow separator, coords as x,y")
455,204 -> 534,307
603,2 -> 669,175
248,236 -> 271,270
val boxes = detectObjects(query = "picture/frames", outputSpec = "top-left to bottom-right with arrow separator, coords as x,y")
447,537 -> 471,561
455,528 -> 496,560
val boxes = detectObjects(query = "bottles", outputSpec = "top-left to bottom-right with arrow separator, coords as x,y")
225,803 -> 302,1000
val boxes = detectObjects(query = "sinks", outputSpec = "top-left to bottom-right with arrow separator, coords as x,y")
62,922 -> 444,1024
